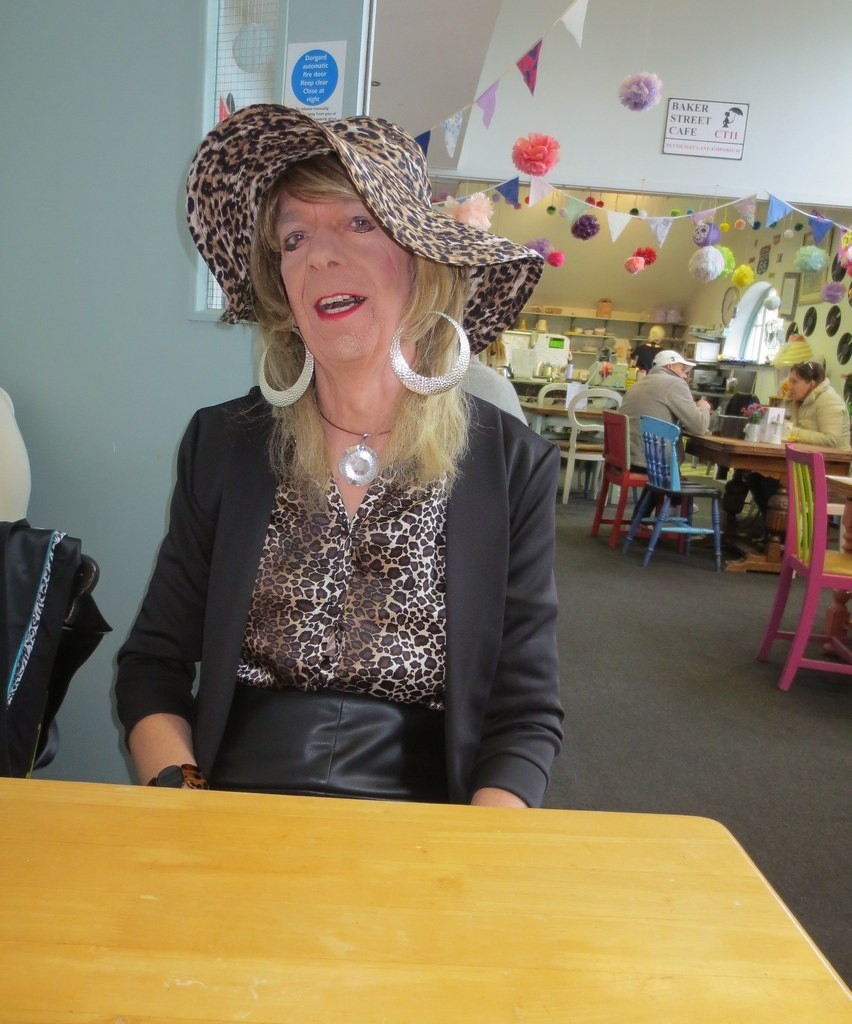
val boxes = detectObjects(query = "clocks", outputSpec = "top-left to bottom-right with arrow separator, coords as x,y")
722,286 -> 740,325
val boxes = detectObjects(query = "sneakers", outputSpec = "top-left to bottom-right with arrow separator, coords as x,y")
668,503 -> 700,518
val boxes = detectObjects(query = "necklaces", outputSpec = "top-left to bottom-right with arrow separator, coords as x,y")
317,394 -> 400,486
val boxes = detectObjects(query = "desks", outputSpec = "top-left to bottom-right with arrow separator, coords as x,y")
680,429 -> 852,574
521,403 -> 605,437
1,779 -> 851,1024
824,474 -> 852,555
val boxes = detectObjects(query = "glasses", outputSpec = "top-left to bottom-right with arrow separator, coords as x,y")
802,360 -> 814,374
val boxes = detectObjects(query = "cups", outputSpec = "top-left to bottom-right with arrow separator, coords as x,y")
566,365 -> 573,379
725,378 -> 738,396
535,320 -> 547,330
534,362 -> 559,378
783,423 -> 793,437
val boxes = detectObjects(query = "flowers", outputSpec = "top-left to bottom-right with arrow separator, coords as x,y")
741,403 -> 766,425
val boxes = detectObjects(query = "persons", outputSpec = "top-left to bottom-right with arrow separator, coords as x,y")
619,350 -> 711,542
629,324 -> 666,373
110,106 -> 564,809
700,361 -> 851,549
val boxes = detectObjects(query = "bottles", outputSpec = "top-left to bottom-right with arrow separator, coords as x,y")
521,318 -> 525,329
768,413 -> 784,444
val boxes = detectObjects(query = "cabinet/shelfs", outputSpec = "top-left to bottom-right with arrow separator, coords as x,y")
565,330 -> 682,357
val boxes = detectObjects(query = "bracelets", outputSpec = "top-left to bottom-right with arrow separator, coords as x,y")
145,764 -> 210,791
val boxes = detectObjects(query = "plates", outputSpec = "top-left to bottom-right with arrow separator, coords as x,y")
570,346 -> 598,352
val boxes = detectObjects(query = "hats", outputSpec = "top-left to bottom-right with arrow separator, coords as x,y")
653,350 -> 696,373
183,102 -> 543,359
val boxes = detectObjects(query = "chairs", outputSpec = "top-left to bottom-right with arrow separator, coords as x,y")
591,411 -> 687,548
759,443 -> 852,689
623,416 -> 723,573
549,388 -> 624,502
536,381 -> 594,435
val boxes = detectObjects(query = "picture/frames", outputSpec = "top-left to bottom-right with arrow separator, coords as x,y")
797,226 -> 832,303
777,272 -> 799,317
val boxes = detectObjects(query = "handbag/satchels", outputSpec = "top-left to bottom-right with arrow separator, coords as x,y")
585,361 -> 628,388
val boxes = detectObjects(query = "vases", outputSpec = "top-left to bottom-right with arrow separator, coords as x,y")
742,424 -> 761,442
